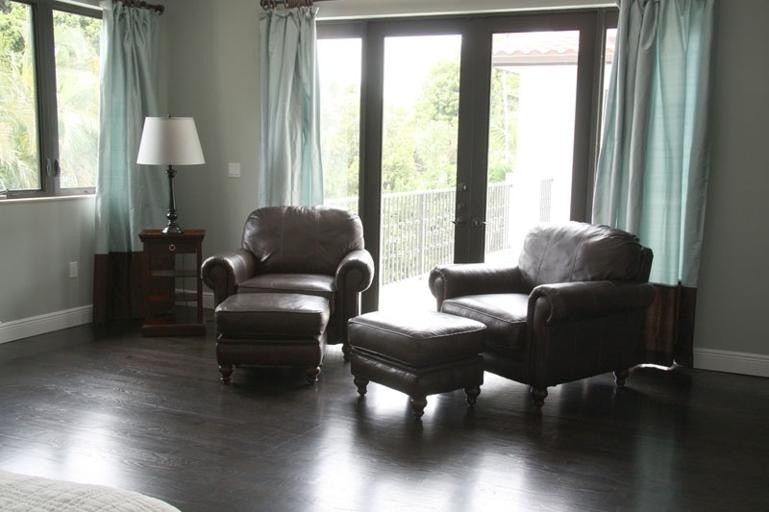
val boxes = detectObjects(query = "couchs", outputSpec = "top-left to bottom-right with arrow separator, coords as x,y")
199,204 -> 375,363
429,220 -> 657,409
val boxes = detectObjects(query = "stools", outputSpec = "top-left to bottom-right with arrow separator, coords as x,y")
345,311 -> 489,421
214,292 -> 331,386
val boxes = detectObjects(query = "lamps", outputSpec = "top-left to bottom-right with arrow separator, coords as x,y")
135,112 -> 206,235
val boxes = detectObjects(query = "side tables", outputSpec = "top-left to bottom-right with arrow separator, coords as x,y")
138,228 -> 206,337
